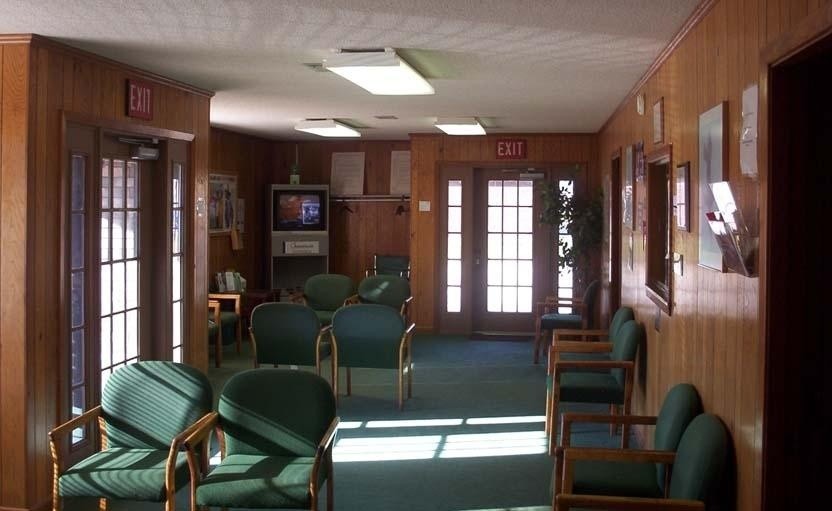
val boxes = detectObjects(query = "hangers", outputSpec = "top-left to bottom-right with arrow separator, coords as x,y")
394,195 -> 405,215
338,198 -> 354,214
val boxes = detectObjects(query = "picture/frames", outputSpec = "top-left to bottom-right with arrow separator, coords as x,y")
698,99 -> 730,273
677,160 -> 690,232
653,96 -> 664,144
621,143 -> 637,230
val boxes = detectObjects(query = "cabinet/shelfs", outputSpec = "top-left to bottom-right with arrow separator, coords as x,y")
553,412 -> 729,510
248,302 -> 332,377
288,274 -> 357,326
546,319 -> 641,455
550,384 -> 704,499
246,289 -> 280,340
553,306 -> 635,373
329,303 -> 415,409
207,294 -> 242,368
534,279 -> 599,365
48,361 -> 216,511
343,275 -> 413,318
182,369 -> 340,510
365,253 -> 411,280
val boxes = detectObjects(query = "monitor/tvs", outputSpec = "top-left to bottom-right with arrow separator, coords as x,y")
273,189 -> 325,232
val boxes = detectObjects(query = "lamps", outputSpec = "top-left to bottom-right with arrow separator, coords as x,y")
432,117 -> 486,136
321,47 -> 436,96
293,118 -> 362,138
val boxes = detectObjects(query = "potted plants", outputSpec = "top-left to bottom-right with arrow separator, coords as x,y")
289,164 -> 300,184
